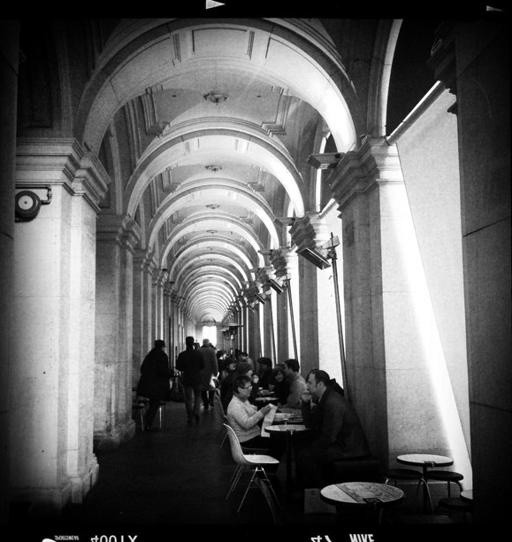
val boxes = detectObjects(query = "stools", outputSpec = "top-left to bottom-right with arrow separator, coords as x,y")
135,388 -> 167,433
376,469 -> 464,541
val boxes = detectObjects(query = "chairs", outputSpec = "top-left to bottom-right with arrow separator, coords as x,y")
212,376 -> 280,512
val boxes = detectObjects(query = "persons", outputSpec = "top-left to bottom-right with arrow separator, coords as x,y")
193,338 -> 200,353
175,336 -> 206,425
195,339 -> 220,410
216,342 -> 369,502
135,339 -> 171,433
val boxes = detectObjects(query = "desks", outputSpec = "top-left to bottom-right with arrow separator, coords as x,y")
321,453 -> 473,525
264,412 -> 311,488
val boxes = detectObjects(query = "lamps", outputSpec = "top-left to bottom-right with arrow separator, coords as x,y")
205,90 -> 227,264
220,323 -> 238,343
